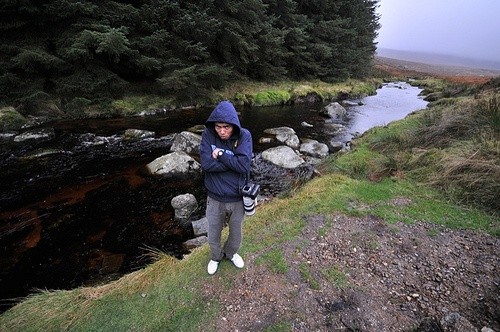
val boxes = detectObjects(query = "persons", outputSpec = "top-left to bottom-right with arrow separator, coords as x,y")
200,100 -> 253,275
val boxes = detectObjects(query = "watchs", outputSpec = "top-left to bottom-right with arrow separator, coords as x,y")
217,148 -> 224,158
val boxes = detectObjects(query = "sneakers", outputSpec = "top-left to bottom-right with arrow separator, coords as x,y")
207,260 -> 219,274
231,253 -> 244,268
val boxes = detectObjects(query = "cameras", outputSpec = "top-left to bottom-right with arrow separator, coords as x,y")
242,184 -> 260,217
